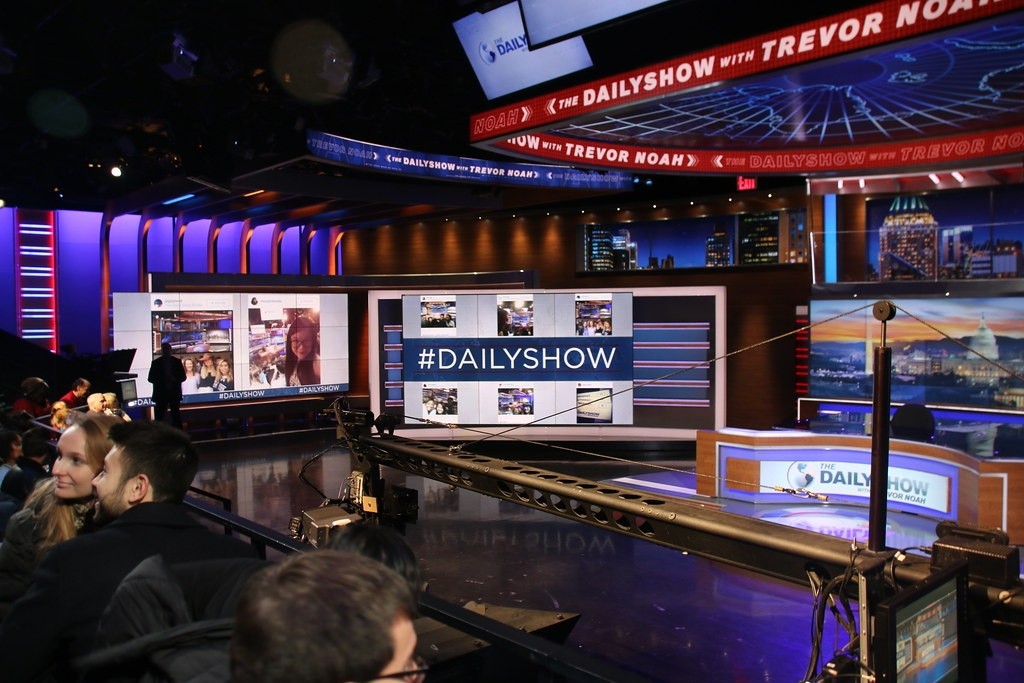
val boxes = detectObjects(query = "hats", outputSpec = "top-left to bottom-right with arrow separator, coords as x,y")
198,354 -> 213,361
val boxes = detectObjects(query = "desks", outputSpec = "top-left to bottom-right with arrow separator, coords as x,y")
696,429 -> 1024,546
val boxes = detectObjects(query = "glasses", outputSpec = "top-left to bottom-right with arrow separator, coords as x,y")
356,655 -> 428,683
290,340 -> 312,349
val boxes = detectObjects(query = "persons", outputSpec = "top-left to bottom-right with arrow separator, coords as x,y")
424,393 -> 459,415
1,378 -> 260,683
228,550 -> 428,683
149,343 -> 186,431
182,351 -> 235,395
576,317 -> 611,335
428,313 -> 455,327
285,317 -> 320,387
498,307 -> 513,336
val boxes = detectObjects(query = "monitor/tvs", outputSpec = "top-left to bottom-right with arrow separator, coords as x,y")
116,379 -> 137,403
874,559 -> 973,683
453,0 -> 667,101
